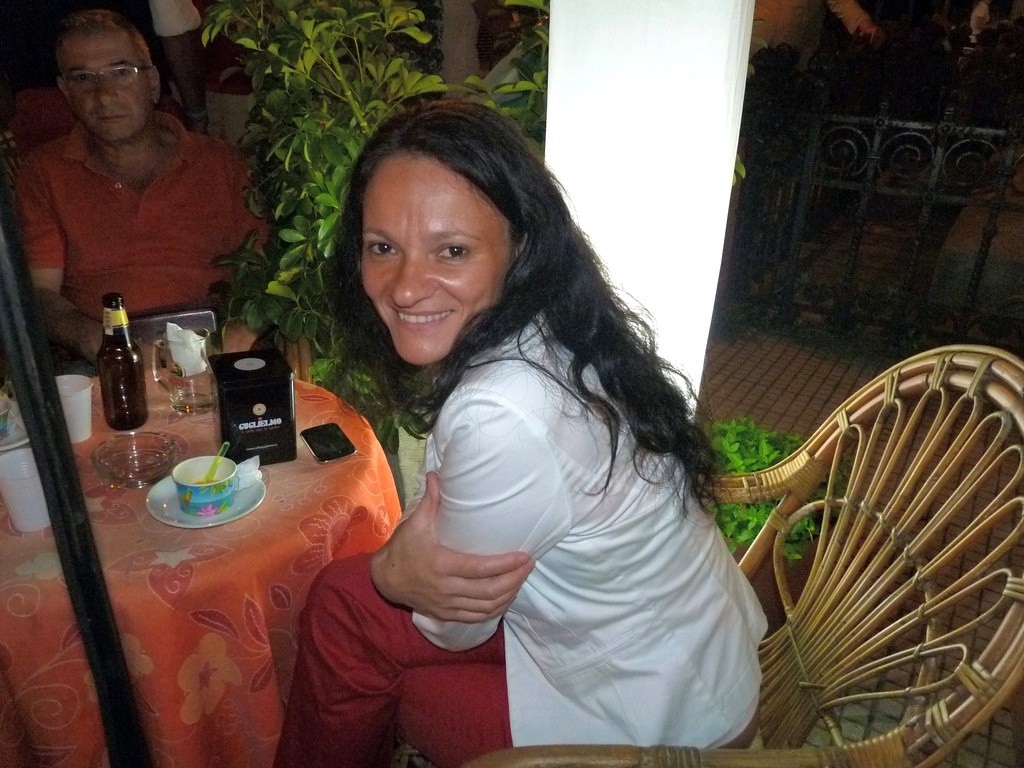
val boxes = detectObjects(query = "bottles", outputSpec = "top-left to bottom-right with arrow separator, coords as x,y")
97,292 -> 149,430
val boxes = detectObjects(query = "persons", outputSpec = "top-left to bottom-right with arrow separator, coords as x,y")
821,0 -> 1024,130
14,16 -> 271,372
128,0 -> 259,146
272,96 -> 772,767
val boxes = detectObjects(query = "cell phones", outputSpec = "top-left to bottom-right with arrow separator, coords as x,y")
128,309 -> 218,351
301,423 -> 357,463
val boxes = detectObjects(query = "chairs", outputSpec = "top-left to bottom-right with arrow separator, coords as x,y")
460,342 -> 1024,768
9,88 -> 185,154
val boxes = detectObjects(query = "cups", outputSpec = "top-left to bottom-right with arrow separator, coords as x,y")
0,448 -> 52,533
56,375 -> 93,444
152,327 -> 217,416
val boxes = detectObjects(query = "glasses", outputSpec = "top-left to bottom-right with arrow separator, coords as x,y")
60,65 -> 156,91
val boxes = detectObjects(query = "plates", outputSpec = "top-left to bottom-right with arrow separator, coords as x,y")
145,475 -> 266,528
0,400 -> 29,453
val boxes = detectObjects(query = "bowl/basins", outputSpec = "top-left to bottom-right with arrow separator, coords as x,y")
0,393 -> 12,441
171,455 -> 238,517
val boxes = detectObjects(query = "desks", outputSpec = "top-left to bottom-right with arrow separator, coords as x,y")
0,360 -> 403,768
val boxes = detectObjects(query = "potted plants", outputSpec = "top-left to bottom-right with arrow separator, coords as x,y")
700,412 -> 858,640
197,0 -> 553,515
